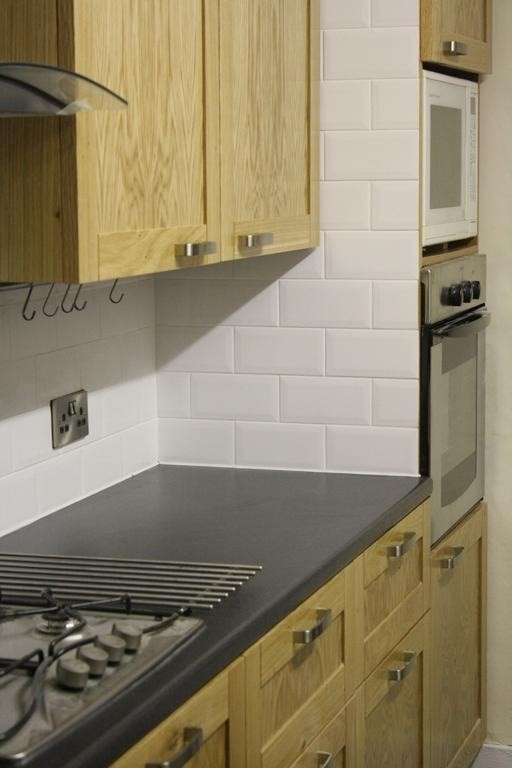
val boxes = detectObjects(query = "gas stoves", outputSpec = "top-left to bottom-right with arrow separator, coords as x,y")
0,547 -> 262,767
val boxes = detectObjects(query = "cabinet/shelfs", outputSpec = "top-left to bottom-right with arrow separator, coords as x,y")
0,0 -> 321,286
353,494 -> 431,767
98,640 -> 243,767
429,496 -> 489,767
420,1 -> 493,77
236,543 -> 357,768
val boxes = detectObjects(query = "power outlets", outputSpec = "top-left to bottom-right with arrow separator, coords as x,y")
50,388 -> 90,450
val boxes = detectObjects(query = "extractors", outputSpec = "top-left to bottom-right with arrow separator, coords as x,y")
0,58 -> 129,121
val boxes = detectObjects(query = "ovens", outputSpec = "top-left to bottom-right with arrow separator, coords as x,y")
417,252 -> 489,545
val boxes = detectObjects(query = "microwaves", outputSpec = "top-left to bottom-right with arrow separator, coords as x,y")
419,60 -> 481,248
